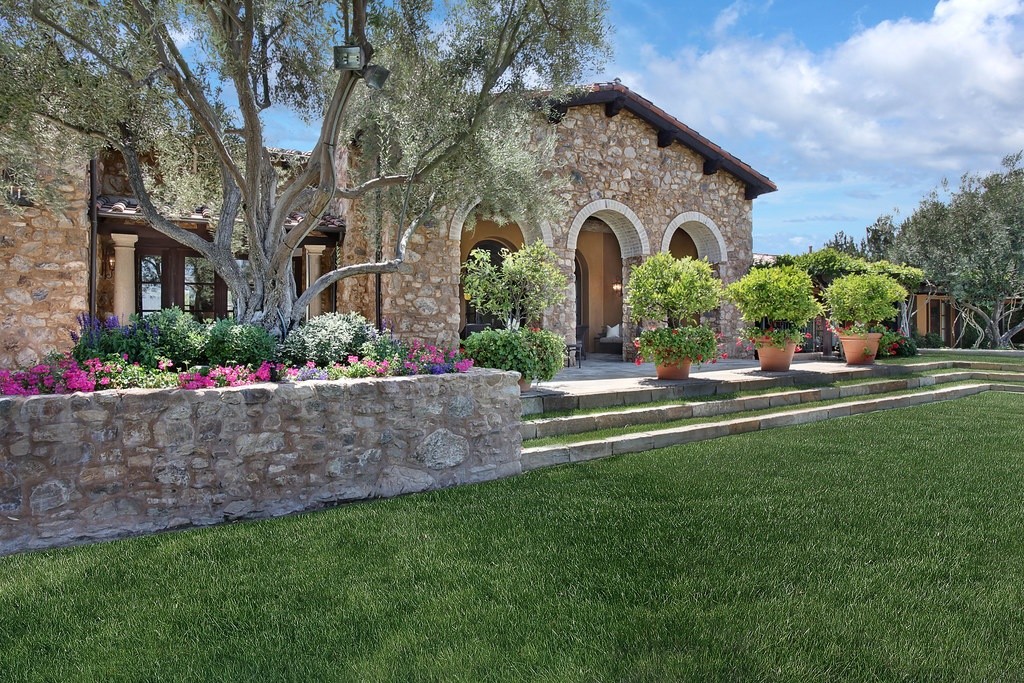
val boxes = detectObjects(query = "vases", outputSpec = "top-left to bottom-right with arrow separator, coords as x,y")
756,339 -> 797,373
837,331 -> 884,367
654,350 -> 692,381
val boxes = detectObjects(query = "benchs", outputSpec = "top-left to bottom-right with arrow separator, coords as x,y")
593,322 -> 623,353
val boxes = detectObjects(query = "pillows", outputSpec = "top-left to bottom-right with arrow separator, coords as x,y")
605,324 -> 620,338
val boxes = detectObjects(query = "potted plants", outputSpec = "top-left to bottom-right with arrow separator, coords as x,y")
460,329 -> 564,392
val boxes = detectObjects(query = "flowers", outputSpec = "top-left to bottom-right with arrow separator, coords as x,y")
826,322 -> 908,360
633,325 -> 727,367
734,326 -> 813,353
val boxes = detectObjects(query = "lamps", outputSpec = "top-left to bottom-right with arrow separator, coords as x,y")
332,43 -> 365,72
358,63 -> 391,92
611,275 -> 622,298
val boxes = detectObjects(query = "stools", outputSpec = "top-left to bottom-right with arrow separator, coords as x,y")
566,343 -> 582,368
